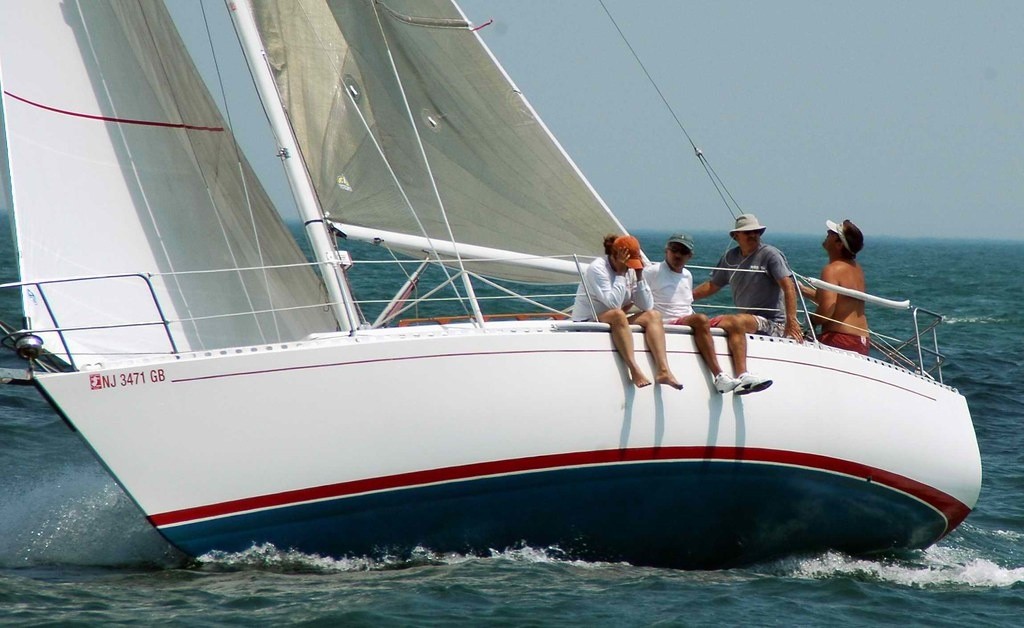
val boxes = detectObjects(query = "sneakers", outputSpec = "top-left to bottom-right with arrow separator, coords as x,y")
714,372 -> 742,394
733,372 -> 773,395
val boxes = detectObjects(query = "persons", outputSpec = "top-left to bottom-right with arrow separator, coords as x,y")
689,213 -> 805,345
640,233 -> 773,395
571,236 -> 683,391
796,218 -> 870,354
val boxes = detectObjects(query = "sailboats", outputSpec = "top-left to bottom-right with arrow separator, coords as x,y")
0,0 -> 981,578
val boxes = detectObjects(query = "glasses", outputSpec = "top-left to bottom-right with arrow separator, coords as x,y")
668,246 -> 690,255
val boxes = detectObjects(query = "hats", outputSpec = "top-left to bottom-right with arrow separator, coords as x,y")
729,214 -> 767,241
612,236 -> 643,270
826,220 -> 862,259
668,231 -> 695,254
